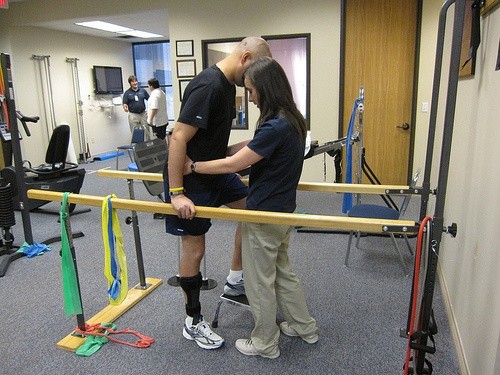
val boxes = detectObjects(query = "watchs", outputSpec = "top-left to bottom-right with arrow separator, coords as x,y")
190,161 -> 197,173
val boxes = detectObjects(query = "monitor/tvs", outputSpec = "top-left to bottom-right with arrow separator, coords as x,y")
92,66 -> 123,94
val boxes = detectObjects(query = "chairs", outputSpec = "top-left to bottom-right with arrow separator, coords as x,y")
115,124 -> 145,170
340,170 -> 421,276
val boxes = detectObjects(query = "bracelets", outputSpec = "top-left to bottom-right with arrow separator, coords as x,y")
169,190 -> 186,196
169,187 -> 184,191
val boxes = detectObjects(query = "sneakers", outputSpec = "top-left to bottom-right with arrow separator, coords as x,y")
234,338 -> 280,359
224,273 -> 245,296
280,322 -> 319,344
182,316 -> 225,349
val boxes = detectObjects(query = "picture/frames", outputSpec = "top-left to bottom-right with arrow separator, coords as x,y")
176,39 -> 193,57
178,79 -> 192,103
176,59 -> 196,78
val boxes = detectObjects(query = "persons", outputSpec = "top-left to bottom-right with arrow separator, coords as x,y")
163,37 -> 272,350
147,78 -> 168,140
122,76 -> 151,144
183,56 -> 319,358
